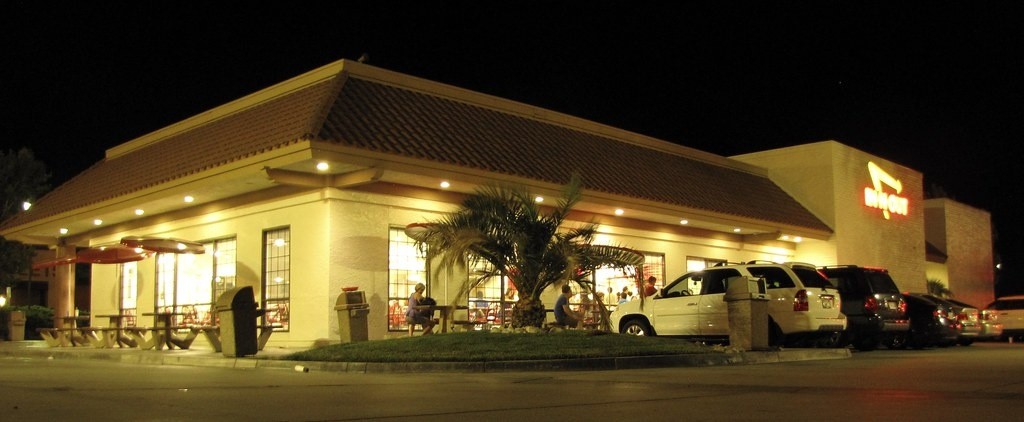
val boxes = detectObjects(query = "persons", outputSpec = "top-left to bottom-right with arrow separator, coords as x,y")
405,282 -> 436,337
496,288 -> 514,318
644,276 -> 658,297
554,285 -> 584,330
471,290 -> 488,317
595,286 -> 638,312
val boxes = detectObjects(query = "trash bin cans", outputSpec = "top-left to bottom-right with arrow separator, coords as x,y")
7,310 -> 26,341
215,286 -> 258,357
333,290 -> 370,344
723,276 -> 770,351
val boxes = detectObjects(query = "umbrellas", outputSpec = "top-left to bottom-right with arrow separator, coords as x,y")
31,255 -> 79,317
76,244 -> 143,315
405,221 -> 459,306
576,244 -> 646,321
121,234 -> 206,314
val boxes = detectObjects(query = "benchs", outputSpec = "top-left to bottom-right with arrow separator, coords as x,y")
36,324 -> 283,352
548,321 -> 600,331
408,318 -> 487,331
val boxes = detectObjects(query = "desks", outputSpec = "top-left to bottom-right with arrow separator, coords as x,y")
51,315 -> 89,347
412,305 -> 469,334
94,315 -> 137,348
143,313 -> 186,350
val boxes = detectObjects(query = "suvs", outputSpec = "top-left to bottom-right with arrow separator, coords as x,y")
817,263 -> 913,351
985,295 -> 1024,343
608,259 -> 848,349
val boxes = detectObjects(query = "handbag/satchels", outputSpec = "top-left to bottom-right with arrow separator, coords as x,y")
419,298 -> 436,304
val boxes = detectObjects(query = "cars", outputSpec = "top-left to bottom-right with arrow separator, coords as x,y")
890,291 -> 962,351
941,296 -> 1003,346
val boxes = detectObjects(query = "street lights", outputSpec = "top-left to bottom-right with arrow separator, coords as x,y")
22,195 -> 34,311
